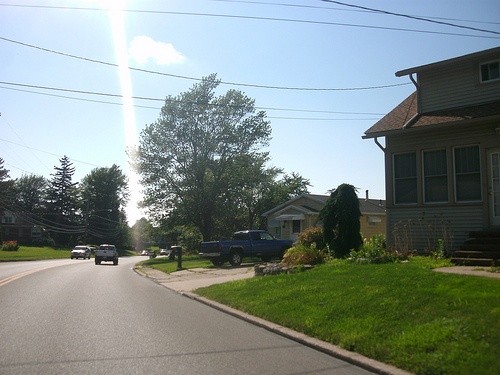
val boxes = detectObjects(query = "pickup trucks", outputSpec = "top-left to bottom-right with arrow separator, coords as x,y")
94,244 -> 119,265
197,229 -> 295,267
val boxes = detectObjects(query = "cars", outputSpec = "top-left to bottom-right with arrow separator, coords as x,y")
70,245 -> 98,259
159,248 -> 170,255
141,250 -> 155,256
